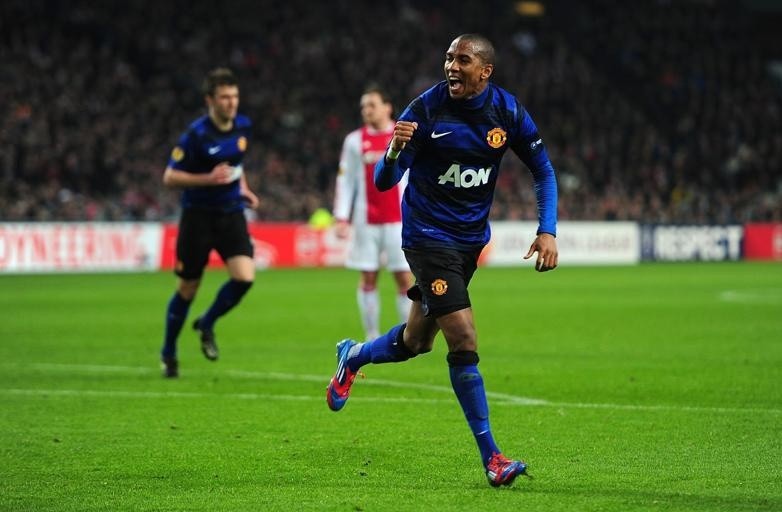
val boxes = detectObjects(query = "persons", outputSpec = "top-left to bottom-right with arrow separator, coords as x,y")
0,1 -> 781,224
333,87 -> 417,344
158,70 -> 262,376
323,34 -> 561,490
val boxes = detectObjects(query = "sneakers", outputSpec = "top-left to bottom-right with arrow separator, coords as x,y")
193,319 -> 218,360
161,350 -> 177,377
485,454 -> 527,488
327,339 -> 357,410
